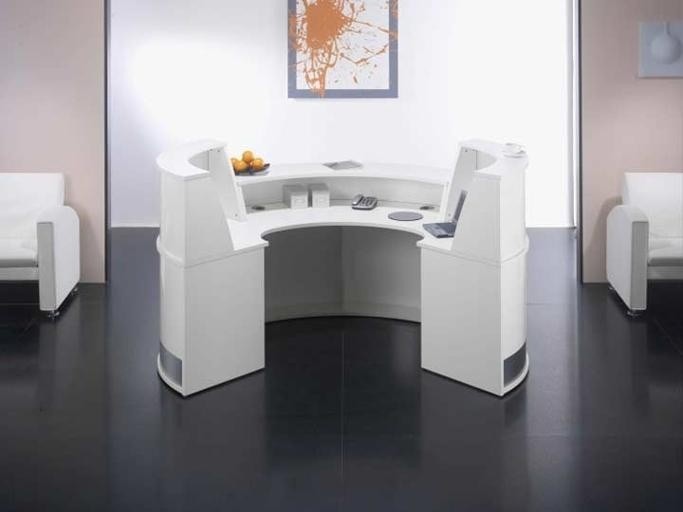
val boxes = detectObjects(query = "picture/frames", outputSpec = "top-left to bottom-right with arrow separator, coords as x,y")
287,0 -> 399,99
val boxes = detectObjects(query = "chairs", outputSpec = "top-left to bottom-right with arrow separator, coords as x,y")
0,171 -> 81,322
604,172 -> 683,321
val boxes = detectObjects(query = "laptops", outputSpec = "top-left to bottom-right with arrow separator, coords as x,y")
423,190 -> 468,238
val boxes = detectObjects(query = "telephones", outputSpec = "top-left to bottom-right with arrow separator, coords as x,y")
352,194 -> 377,210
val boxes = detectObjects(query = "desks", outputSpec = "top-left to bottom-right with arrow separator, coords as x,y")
155,140 -> 530,399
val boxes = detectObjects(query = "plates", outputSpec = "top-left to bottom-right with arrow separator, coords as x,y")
228,160 -> 271,176
501,150 -> 527,159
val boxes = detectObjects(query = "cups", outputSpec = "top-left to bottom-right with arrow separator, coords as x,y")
505,142 -> 521,156
